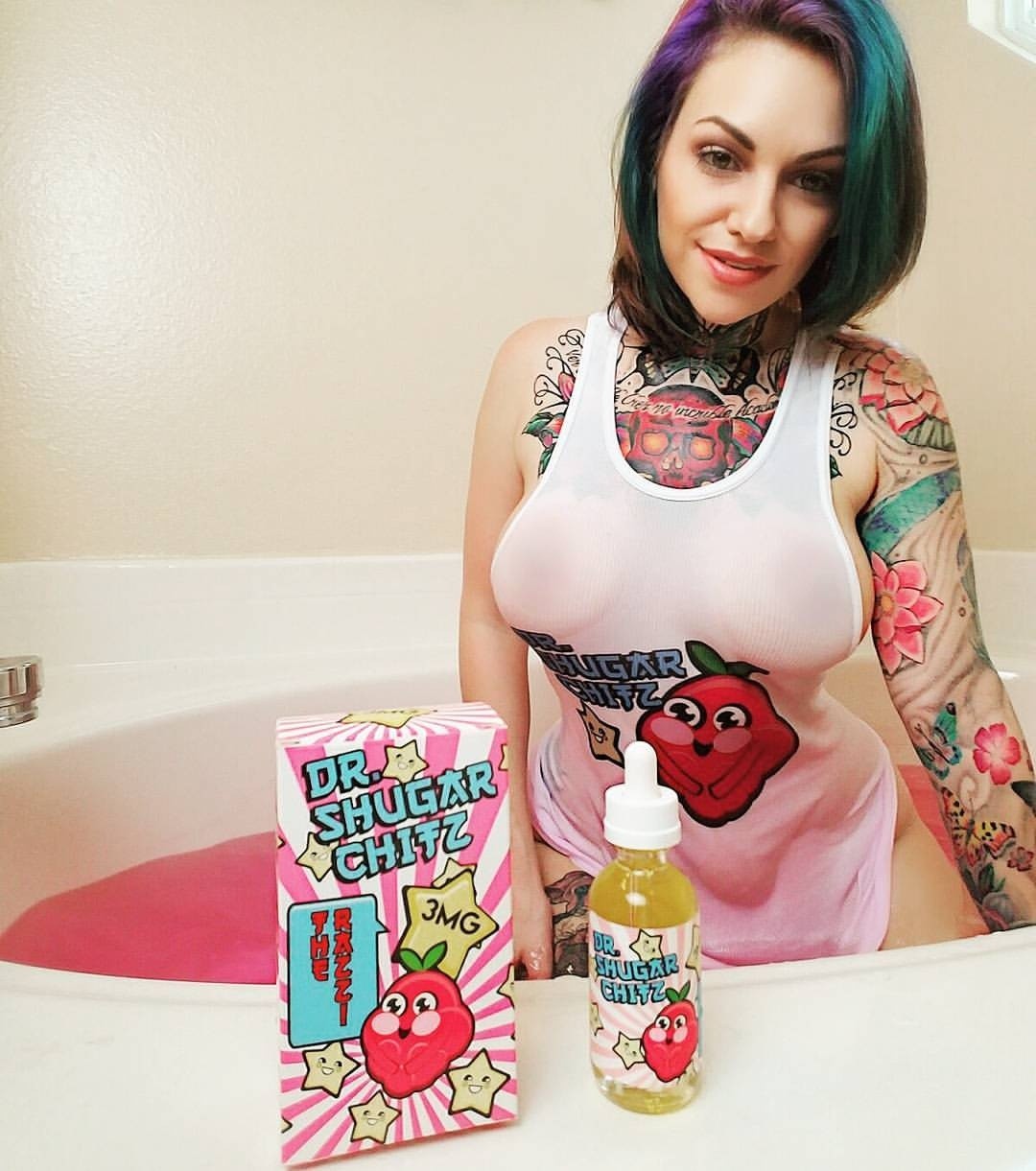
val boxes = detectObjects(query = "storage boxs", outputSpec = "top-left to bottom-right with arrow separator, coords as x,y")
272,703 -> 520,1168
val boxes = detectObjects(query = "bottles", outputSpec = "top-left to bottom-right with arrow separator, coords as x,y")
588,743 -> 702,1115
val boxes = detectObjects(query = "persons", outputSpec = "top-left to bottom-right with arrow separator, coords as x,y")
459,0 -> 1036,981
